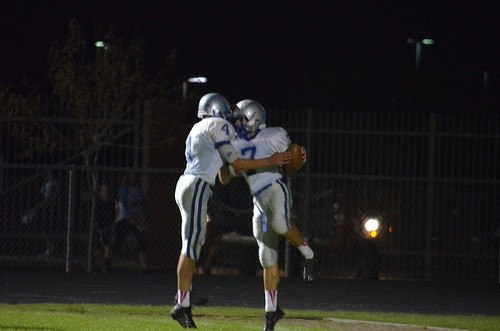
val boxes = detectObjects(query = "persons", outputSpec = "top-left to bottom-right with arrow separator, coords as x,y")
170,93 -> 292,328
219,99 -> 319,331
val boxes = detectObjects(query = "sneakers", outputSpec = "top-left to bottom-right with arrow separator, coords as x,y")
304,251 -> 318,284
263,306 -> 285,331
173,293 -> 179,305
171,304 -> 197,329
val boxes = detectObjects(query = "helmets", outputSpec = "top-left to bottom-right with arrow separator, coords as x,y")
231,100 -> 266,132
197,93 -> 232,121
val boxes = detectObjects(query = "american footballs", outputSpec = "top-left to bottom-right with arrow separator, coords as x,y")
286,144 -> 306,174
